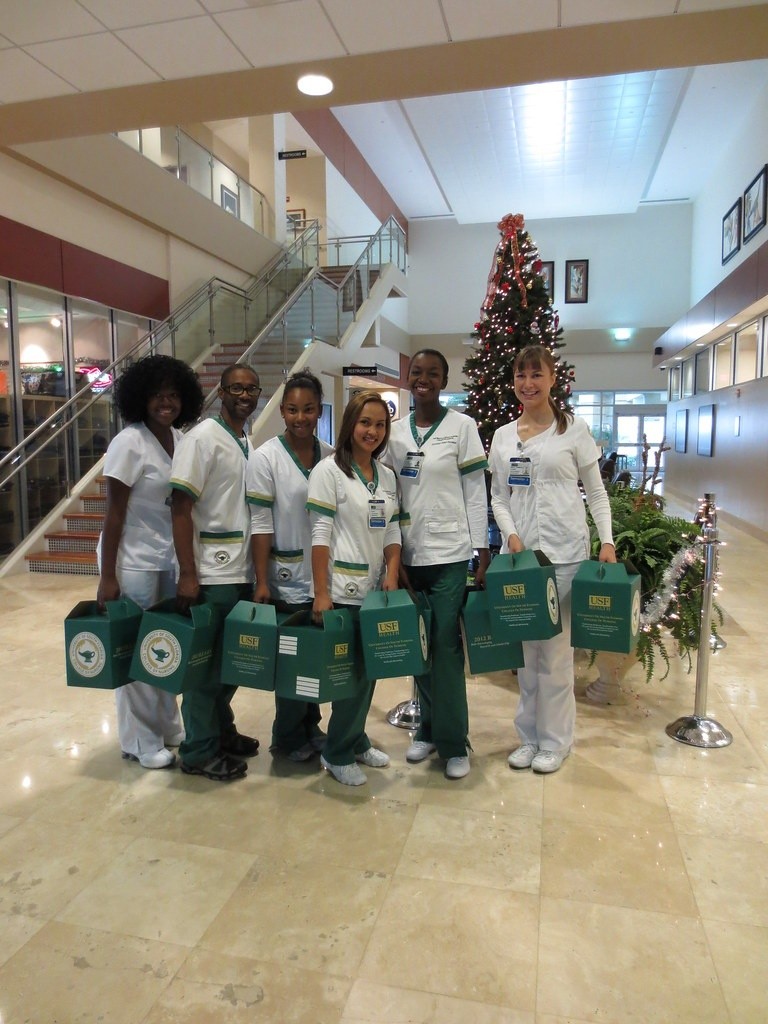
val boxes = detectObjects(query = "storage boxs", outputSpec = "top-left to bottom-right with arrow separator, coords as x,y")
64,552 -> 641,702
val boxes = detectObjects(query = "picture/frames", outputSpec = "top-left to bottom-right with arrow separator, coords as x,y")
742,163 -> 768,246
721,197 -> 742,265
287,209 -> 306,231
535,261 -> 554,304
220,184 -> 240,217
565,259 -> 587,303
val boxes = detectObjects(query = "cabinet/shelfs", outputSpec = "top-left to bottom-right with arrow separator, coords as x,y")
0,394 -> 110,556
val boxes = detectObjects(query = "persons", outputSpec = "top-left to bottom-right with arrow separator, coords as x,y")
170,364 -> 258,781
308,389 -> 401,788
487,345 -> 619,774
246,369 -> 336,763
97,355 -> 204,769
376,349 -> 488,780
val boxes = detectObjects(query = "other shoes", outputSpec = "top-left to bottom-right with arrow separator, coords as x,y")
269,730 -> 328,761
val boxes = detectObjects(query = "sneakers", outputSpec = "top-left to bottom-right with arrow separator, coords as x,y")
354,747 -> 389,767
165,730 -> 186,746
219,733 -> 259,754
406,740 -> 436,760
121,746 -> 176,768
446,746 -> 470,778
320,754 -> 368,786
531,746 -> 571,773
508,742 -> 538,769
180,754 -> 248,780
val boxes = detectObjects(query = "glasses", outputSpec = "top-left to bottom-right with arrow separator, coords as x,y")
223,383 -> 262,397
